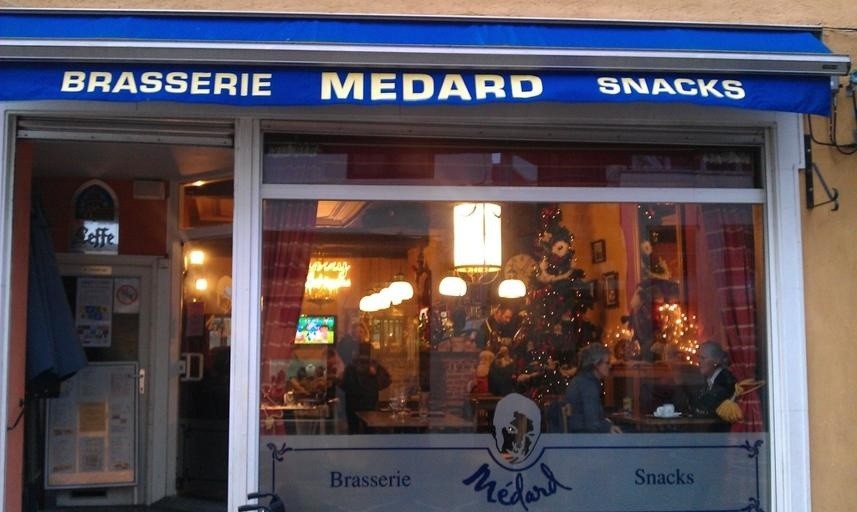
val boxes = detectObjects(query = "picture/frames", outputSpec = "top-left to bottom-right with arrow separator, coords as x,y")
602,271 -> 619,308
592,239 -> 606,264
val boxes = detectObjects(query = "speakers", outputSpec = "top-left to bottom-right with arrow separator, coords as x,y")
132,180 -> 163,199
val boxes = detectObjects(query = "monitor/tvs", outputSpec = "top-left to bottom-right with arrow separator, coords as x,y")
293,315 -> 336,346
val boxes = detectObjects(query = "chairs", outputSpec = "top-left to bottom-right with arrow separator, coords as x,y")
545,402 -> 571,432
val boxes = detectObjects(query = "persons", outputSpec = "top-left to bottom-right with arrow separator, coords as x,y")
281,323 -> 392,436
474,300 -> 623,435
675,341 -> 737,432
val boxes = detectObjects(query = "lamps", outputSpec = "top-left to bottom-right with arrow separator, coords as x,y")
359,202 -> 527,313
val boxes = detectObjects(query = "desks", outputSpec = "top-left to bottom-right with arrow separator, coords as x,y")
355,411 -> 474,434
614,412 -> 716,432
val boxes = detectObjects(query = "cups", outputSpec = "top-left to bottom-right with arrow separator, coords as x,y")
657,403 -> 676,416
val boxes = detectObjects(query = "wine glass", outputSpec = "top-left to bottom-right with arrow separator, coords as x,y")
388,391 -> 410,420
417,391 -> 431,424
